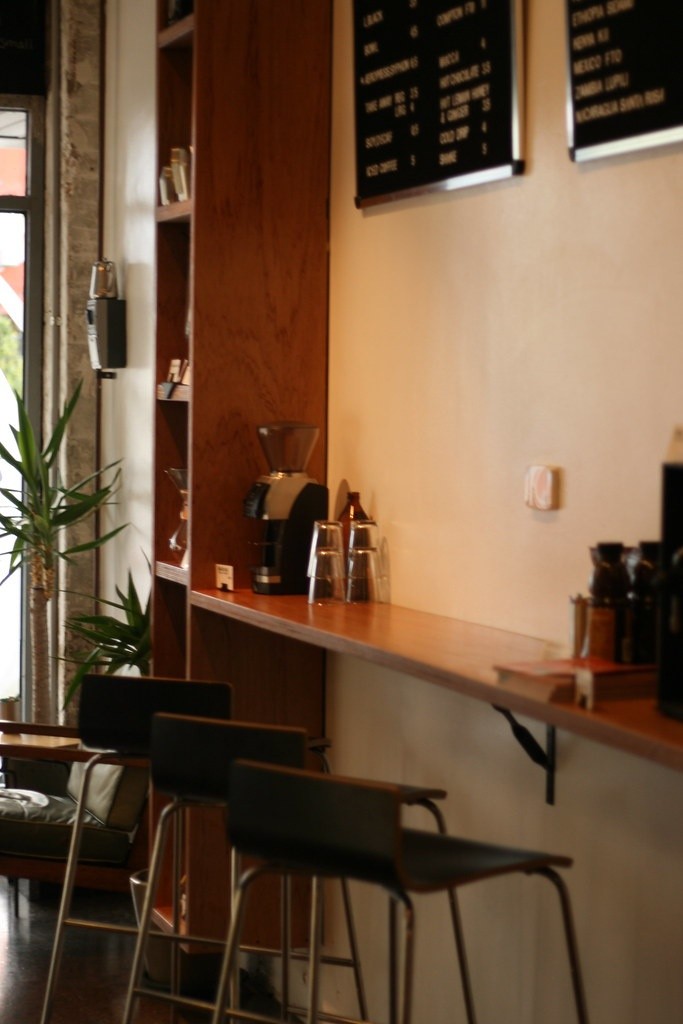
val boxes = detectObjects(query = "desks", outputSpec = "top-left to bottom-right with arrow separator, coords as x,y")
192,586 -> 683,806
0,733 -> 80,796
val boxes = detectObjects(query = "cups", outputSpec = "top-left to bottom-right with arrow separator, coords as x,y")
88,258 -> 118,299
307,519 -> 381,605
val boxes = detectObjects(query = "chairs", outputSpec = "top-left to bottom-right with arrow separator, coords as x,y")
119,712 -> 331,1024
37,674 -> 235,1024
211,759 -> 590,1024
0,720 -> 148,919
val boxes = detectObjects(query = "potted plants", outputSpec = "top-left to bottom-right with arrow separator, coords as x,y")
0,696 -> 23,734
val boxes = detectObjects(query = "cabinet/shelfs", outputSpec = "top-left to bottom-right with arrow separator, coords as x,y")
144,0 -> 330,953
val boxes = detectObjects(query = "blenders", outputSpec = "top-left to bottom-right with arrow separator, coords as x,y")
243,423 -> 329,596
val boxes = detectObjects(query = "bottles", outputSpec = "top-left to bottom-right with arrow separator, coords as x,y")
159,467 -> 188,564
